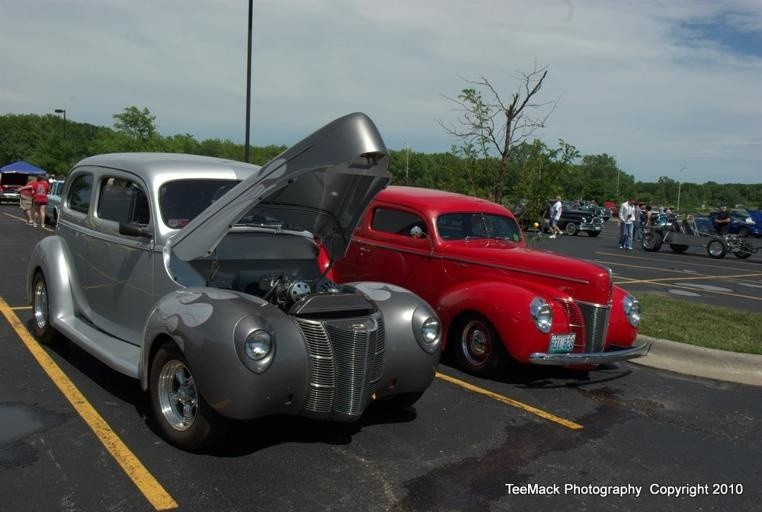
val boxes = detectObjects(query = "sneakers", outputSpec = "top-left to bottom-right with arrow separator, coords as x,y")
619,245 -> 633,250
548,231 -> 564,239
25,220 -> 45,228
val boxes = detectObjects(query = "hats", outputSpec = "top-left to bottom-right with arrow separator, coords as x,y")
410,226 -> 422,236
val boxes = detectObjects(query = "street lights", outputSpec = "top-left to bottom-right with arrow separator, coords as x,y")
54,108 -> 67,140
676,165 -> 688,211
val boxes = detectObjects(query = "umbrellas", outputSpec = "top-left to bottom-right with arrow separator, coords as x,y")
0,161 -> 47,175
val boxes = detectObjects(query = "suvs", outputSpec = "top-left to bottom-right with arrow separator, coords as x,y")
25,108 -> 446,454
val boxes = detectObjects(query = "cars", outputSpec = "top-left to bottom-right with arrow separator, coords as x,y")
314,183 -> 652,382
0,171 -> 28,203
44,179 -> 66,225
508,198 -> 762,260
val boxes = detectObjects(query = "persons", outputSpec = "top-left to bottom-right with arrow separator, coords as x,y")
537,200 -> 554,238
663,208 -> 675,222
714,202 -> 731,236
49,174 -> 57,184
619,196 -> 652,249
549,195 -> 564,239
19,175 -> 50,228
